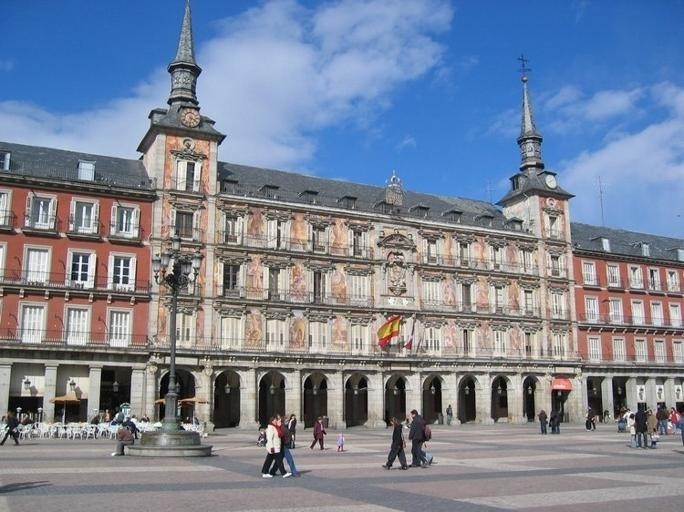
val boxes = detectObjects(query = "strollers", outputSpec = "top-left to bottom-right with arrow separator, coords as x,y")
256,425 -> 267,447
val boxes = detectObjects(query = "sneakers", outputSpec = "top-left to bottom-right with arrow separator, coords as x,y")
402,457 -> 433,470
383,464 -> 390,470
262,472 -> 300,479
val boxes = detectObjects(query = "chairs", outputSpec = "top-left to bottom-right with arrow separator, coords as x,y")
1,422 -> 211,442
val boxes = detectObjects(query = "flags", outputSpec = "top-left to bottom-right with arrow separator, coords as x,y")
402,314 -> 415,349
376,315 -> 400,346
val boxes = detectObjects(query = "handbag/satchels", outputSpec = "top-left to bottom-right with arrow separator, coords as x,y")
425,426 -> 432,441
402,439 -> 406,448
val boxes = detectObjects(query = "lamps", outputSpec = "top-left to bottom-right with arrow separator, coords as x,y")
312,383 -> 320,394
352,385 -> 359,395
392,385 -> 398,395
430,382 -> 436,394
22,378 -> 30,389
528,382 -> 533,394
497,383 -> 502,395
616,386 -> 622,395
176,375 -> 180,393
225,375 -> 231,393
69,377 -> 75,391
592,388 -> 597,395
113,369 -> 121,391
464,386 -> 470,395
269,376 -> 276,395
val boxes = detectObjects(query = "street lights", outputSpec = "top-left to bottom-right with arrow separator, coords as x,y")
150,228 -> 204,430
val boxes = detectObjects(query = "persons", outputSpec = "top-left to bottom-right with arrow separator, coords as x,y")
0,411 -> 29,446
183,417 -> 191,423
310,417 -> 327,450
90,407 -> 150,456
585,402 -> 684,450
194,417 -> 199,425
382,410 -> 433,470
446,405 -> 452,425
337,432 -> 344,452
256,411 -> 297,479
539,407 -> 561,434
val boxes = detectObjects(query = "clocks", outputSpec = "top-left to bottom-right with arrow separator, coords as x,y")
180,109 -> 199,128
545,174 -> 557,189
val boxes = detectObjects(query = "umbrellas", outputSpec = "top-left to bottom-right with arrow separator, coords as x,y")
155,399 -> 181,403
49,395 -> 81,425
180,397 -> 209,414
